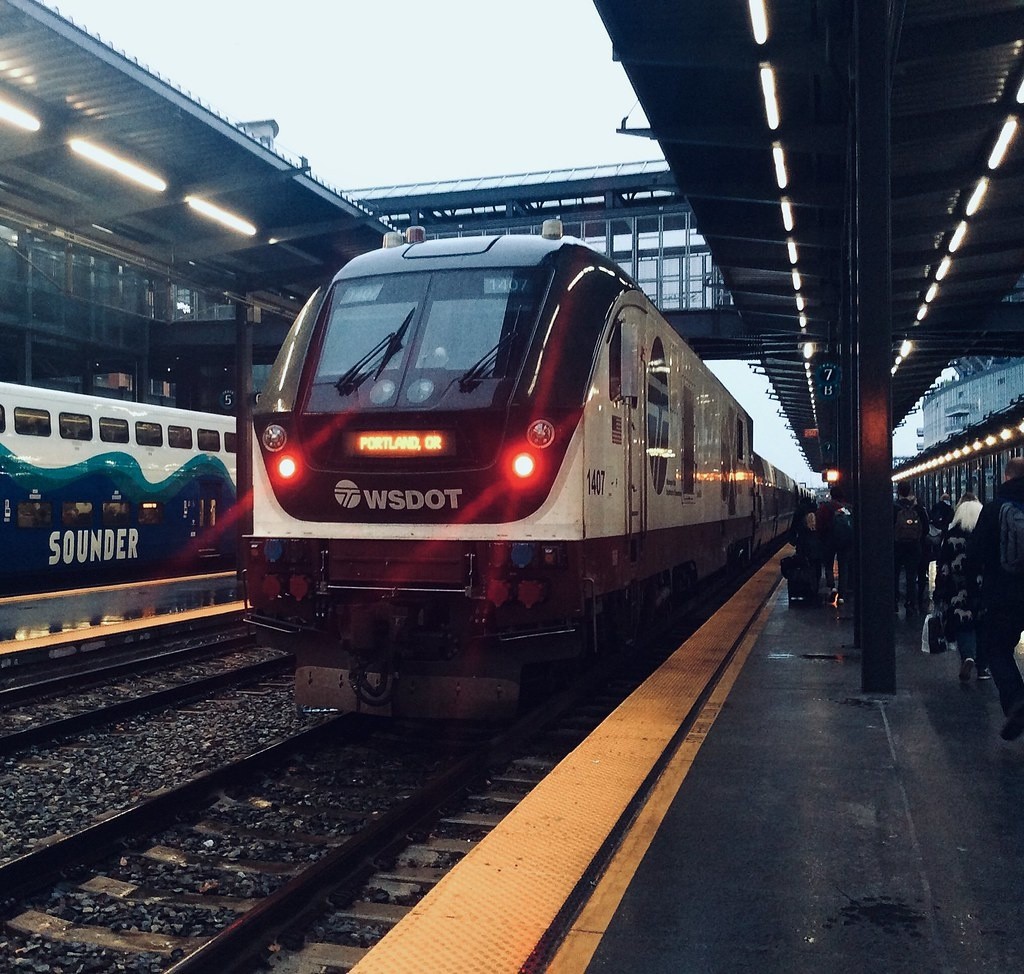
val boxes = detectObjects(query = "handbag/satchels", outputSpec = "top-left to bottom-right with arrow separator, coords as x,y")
781,555 -> 795,580
921,614 -> 948,655
923,506 -> 942,547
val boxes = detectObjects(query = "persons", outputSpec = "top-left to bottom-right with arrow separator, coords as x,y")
13,500 -> 161,528
780,455 -> 1024,742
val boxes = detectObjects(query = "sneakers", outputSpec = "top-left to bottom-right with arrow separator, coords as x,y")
959,656 -> 975,684
976,668 -> 992,679
827,587 -> 838,604
837,595 -> 847,605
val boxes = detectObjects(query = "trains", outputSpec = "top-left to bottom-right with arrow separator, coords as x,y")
0,381 -> 237,637
236,215 -> 804,723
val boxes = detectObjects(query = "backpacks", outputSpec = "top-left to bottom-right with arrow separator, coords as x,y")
822,501 -> 855,542
995,497 -> 1024,577
893,504 -> 922,544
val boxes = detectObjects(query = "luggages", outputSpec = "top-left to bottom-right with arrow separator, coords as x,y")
788,555 -> 821,606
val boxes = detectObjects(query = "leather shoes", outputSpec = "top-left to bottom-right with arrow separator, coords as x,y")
1000,699 -> 1024,741
